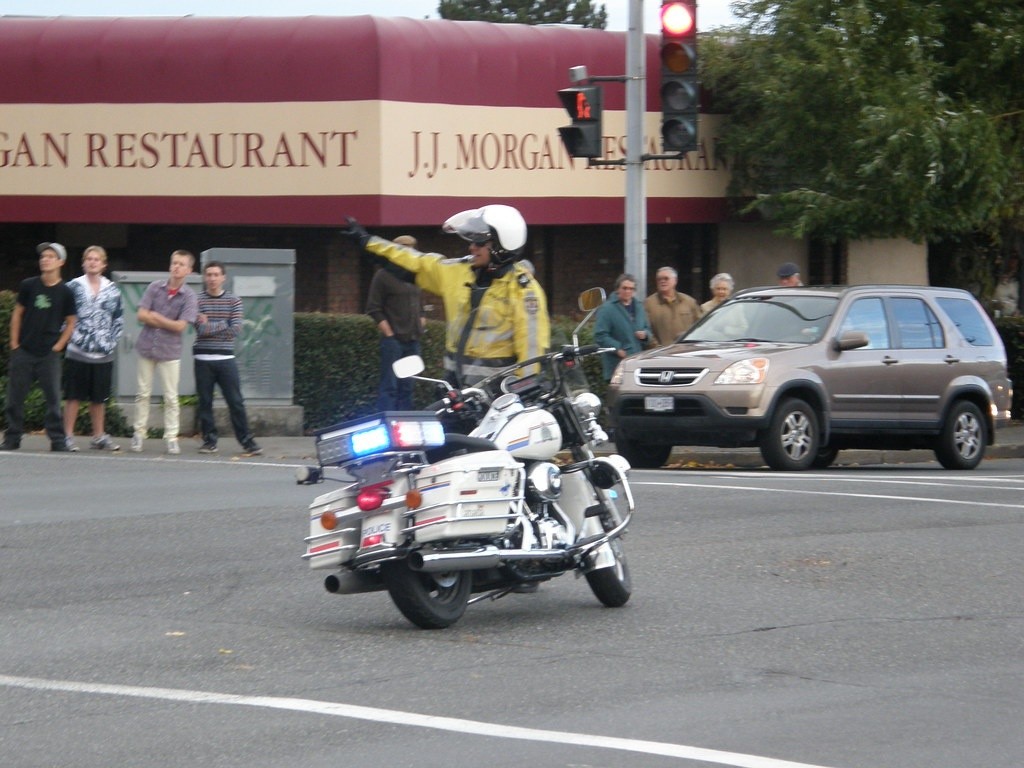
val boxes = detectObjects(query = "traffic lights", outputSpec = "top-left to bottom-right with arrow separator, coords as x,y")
557,86 -> 603,158
659,0 -> 700,152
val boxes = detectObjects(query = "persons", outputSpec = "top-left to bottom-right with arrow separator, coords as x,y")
0,241 -> 77,451
193,261 -> 264,455
336,204 -> 551,593
776,263 -> 803,287
700,273 -> 748,339
130,249 -> 199,454
644,266 -> 704,349
60,246 -> 124,451
990,250 -> 1024,320
366,235 -> 427,411
593,273 -> 652,384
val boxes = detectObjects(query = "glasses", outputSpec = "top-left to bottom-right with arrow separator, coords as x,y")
468,237 -> 494,248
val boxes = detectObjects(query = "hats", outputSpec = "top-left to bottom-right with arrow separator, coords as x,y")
394,236 -> 417,246
37,242 -> 67,259
776,264 -> 800,276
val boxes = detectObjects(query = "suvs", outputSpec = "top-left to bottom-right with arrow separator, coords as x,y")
601,284 -> 1013,471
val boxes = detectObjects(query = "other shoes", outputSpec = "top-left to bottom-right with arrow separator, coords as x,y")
0,440 -> 19,449
52,443 -> 69,451
243,439 -> 263,455
130,432 -> 143,451
89,434 -> 120,450
198,440 -> 218,452
168,439 -> 179,453
65,435 -> 80,451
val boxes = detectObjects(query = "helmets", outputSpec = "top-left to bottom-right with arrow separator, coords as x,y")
442,205 -> 527,264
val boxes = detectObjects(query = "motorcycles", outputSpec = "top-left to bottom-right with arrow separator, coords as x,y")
296,287 -> 636,629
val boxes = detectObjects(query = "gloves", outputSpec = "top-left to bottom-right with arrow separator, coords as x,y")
340,216 -> 371,244
